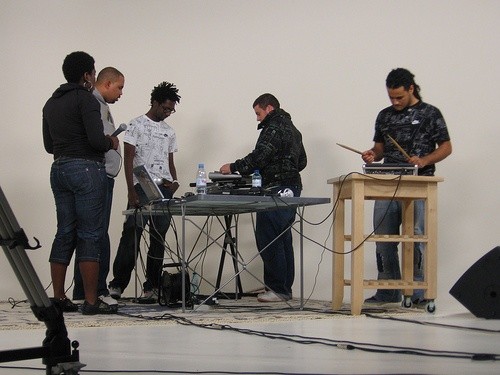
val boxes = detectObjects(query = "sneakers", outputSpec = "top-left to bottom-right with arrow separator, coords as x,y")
82,298 -> 119,315
60,297 -> 78,312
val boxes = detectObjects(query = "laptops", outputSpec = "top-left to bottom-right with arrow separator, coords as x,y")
132,163 -> 177,201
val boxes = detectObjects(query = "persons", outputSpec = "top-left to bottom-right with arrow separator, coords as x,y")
43,51 -> 117,315
362,68 -> 452,305
108,81 -> 181,300
71,67 -> 125,307
221,93 -> 307,303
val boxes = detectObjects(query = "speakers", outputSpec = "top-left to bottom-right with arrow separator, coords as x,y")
448,246 -> 500,319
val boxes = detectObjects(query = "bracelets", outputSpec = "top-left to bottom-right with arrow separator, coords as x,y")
172,180 -> 178,184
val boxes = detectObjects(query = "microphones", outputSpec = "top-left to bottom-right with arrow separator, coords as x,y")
111,123 -> 127,136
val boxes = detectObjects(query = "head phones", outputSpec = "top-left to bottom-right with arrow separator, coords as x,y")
279,188 -> 294,197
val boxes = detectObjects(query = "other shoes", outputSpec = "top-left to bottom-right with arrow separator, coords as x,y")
109,287 -> 122,299
364,295 -> 402,307
257,290 -> 287,302
71,299 -> 85,309
411,296 -> 428,309
144,289 -> 159,299
98,294 -> 125,307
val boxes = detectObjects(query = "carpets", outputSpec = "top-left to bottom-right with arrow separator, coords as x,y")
0,290 -> 425,332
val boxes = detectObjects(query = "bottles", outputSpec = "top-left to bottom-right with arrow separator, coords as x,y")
196,163 -> 206,195
252,170 -> 261,195
191,273 -> 199,295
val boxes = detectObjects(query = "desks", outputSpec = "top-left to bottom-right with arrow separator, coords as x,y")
327,174 -> 444,317
122,195 -> 332,309
